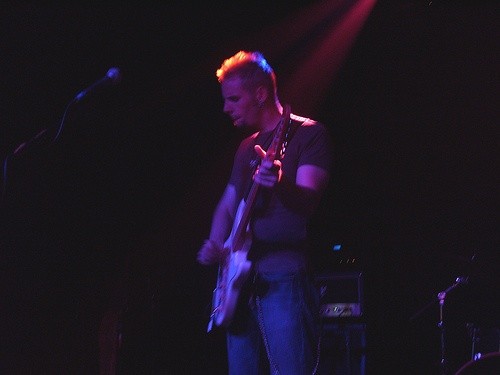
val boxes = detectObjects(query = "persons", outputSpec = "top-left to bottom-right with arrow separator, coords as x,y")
198,49 -> 332,375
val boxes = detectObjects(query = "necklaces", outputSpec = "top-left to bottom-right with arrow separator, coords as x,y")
242,120 -> 280,175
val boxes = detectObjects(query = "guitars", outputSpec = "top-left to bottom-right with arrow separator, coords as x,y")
205,104 -> 292,336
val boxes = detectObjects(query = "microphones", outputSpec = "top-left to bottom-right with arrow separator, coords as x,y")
438,275 -> 468,299
76,68 -> 119,99
